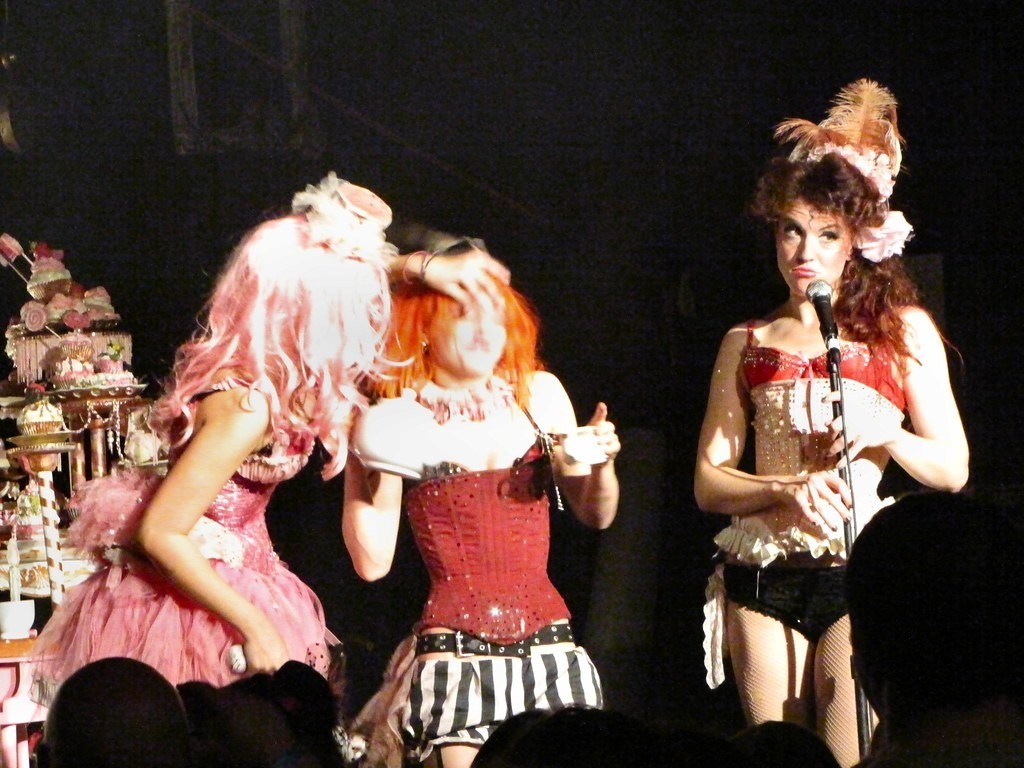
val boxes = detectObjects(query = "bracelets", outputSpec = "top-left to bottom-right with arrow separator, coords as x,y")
419,253 -> 437,278
402,250 -> 425,284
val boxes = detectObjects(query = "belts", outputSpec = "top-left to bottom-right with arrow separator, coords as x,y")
415,624 -> 576,658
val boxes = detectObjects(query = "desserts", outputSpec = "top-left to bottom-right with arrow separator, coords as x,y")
0,241 -> 134,438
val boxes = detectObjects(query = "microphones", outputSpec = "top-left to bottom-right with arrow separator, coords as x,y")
806,280 -> 843,364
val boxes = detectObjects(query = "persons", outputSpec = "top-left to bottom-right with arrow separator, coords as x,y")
694,156 -> 969,768
469,705 -> 843,768
29,170 -> 511,706
842,490 -> 1024,768
47,657 -> 345,767
342,234 -> 621,768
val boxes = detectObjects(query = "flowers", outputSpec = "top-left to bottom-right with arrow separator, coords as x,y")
853,210 -> 914,264
97,339 -> 125,361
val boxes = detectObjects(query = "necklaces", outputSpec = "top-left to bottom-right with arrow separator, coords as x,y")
418,375 -> 512,424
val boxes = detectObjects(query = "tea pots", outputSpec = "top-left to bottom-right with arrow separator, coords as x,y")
345,387 -> 448,480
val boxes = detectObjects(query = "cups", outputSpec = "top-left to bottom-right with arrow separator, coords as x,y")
0,600 -> 35,639
563,427 -> 608,465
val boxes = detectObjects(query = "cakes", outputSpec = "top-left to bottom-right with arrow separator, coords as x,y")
0,474 -> 139,599
